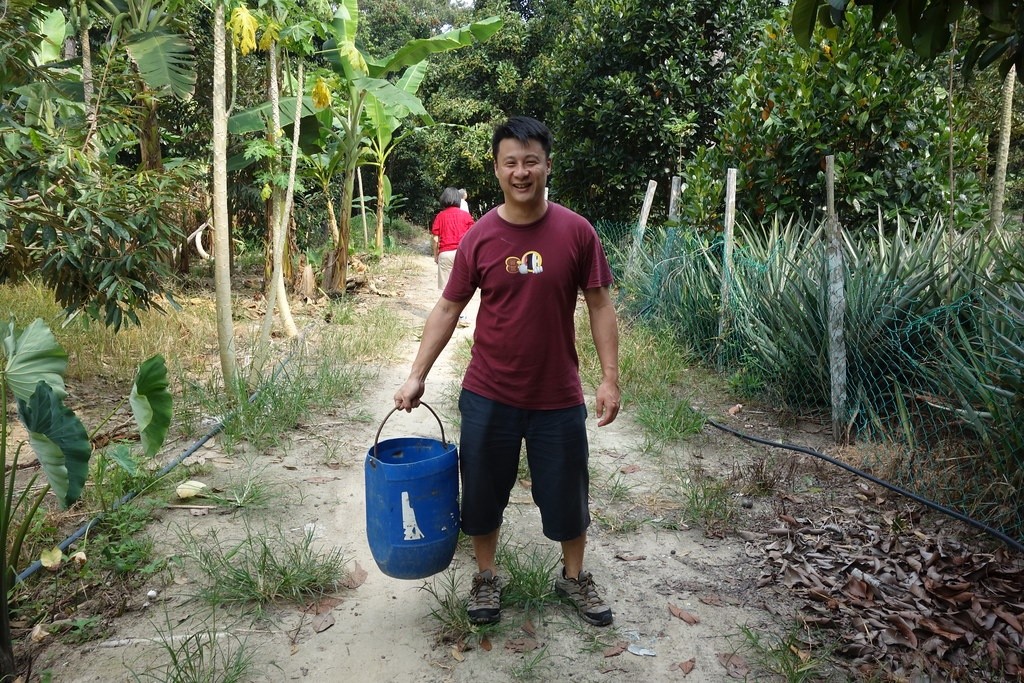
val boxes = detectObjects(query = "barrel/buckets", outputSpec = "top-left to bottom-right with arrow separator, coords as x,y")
364,399 -> 460,580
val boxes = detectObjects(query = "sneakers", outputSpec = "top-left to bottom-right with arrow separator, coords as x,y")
554,566 -> 613,626
467,570 -> 502,624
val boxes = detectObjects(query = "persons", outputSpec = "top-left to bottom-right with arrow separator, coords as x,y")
393,116 -> 624,627
431,186 -> 476,325
457,189 -> 470,214
